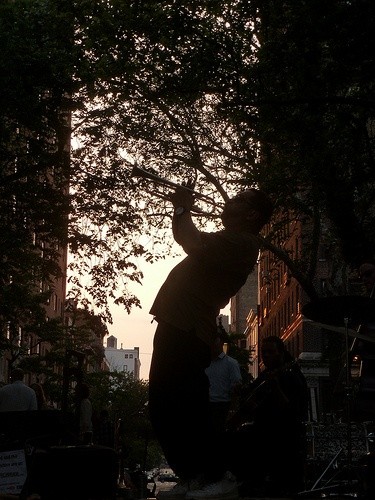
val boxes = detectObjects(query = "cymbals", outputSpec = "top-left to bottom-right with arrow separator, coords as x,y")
301,294 -> 375,327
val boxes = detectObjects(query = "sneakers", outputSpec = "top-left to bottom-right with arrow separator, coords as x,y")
156,474 -> 212,500
183,470 -> 240,500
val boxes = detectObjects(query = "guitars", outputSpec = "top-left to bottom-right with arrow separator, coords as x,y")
301,320 -> 375,347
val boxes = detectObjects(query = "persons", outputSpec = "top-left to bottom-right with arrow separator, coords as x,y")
353,257 -> 375,419
146,188 -> 273,500
30,384 -> 45,411
0,368 -> 37,411
73,383 -> 92,444
205,323 -> 309,500
97,410 -> 115,431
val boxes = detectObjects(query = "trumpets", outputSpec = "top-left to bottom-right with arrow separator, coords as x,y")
131,163 -> 225,217
